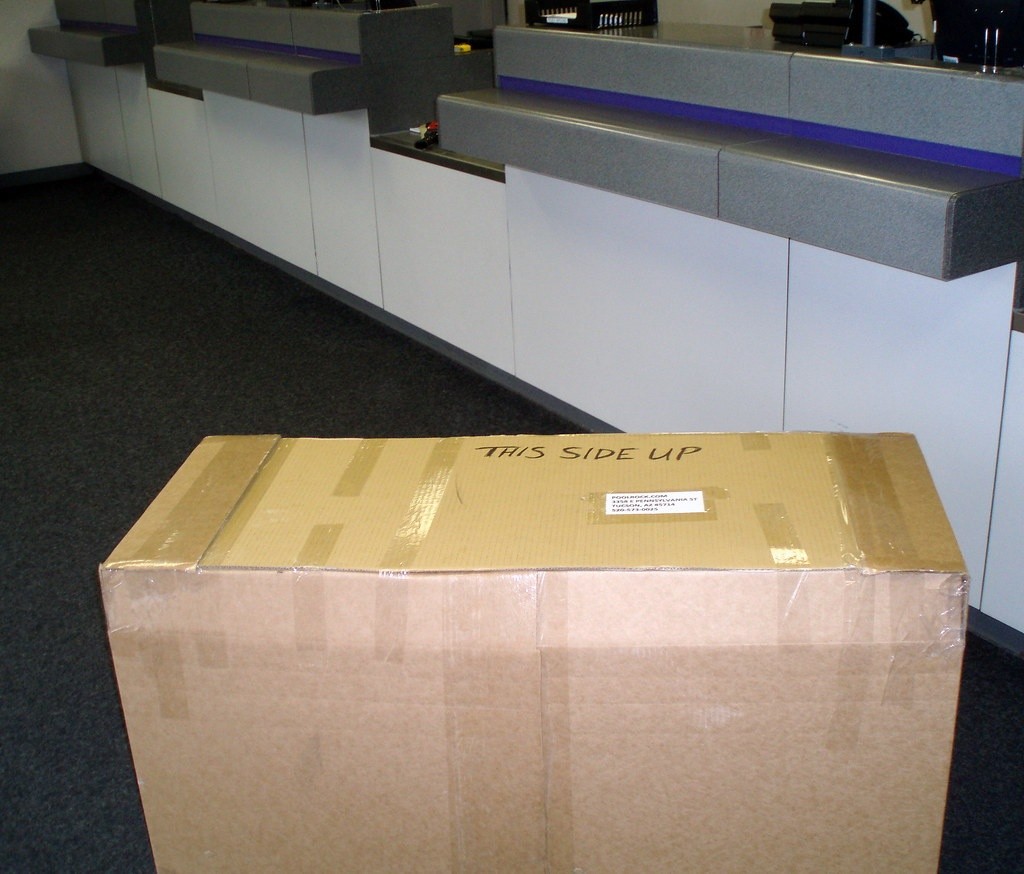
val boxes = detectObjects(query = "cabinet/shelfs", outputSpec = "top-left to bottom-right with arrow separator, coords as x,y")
26,0 -> 1024,661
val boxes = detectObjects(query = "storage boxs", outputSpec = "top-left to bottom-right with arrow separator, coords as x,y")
95,430 -> 972,874
